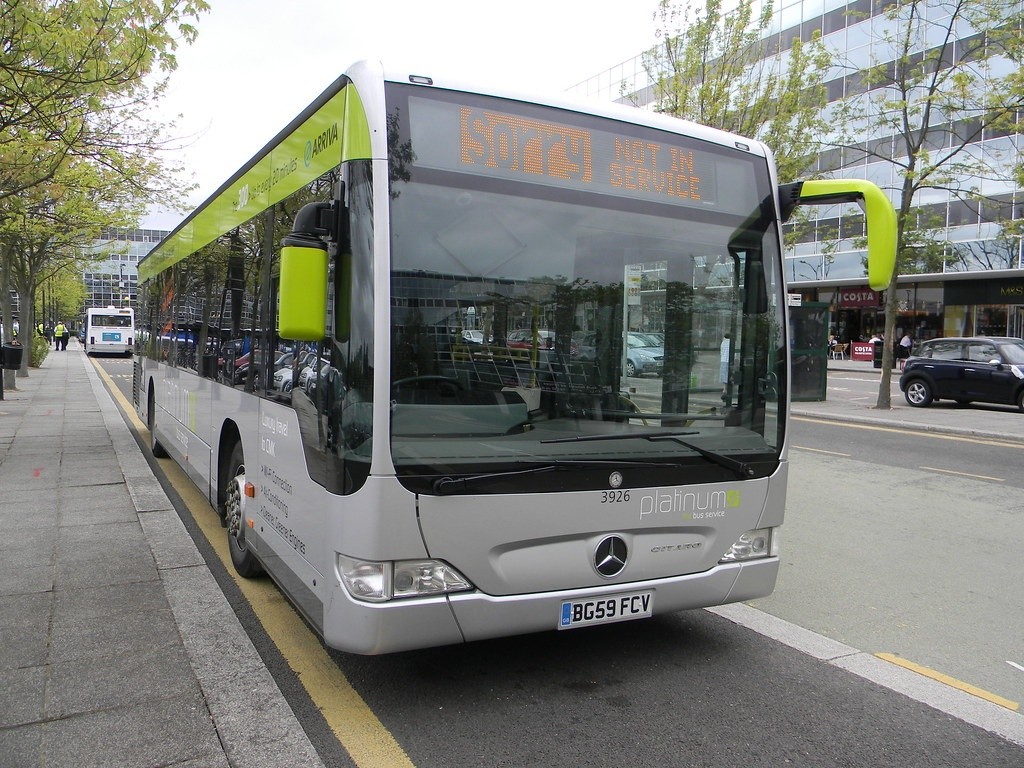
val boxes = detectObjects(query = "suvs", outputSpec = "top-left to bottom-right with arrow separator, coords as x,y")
899,336 -> 1024,413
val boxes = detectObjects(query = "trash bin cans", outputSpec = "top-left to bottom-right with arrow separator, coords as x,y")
203,354 -> 218,382
2,341 -> 23,370
873,341 -> 897,369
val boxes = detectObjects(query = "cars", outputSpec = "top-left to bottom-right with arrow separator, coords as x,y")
165,333 -> 347,401
459,322 -> 665,377
76,327 -> 85,343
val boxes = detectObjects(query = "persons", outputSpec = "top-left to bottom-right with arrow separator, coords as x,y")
12,319 -> 52,341
719,332 -> 730,395
898,331 -> 915,358
53,321 -> 69,351
828,330 -> 884,358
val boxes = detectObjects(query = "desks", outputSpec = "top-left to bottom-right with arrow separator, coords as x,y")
829,345 -> 835,360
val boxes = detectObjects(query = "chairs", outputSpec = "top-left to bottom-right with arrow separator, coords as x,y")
833,344 -> 844,360
843,343 -> 849,359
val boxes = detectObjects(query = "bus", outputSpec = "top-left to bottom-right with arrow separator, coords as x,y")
83,305 -> 135,357
129,55 -> 900,658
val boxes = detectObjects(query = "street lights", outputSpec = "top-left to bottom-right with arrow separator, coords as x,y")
799,259 -> 835,280
120,263 -> 127,309
904,289 -> 912,312
